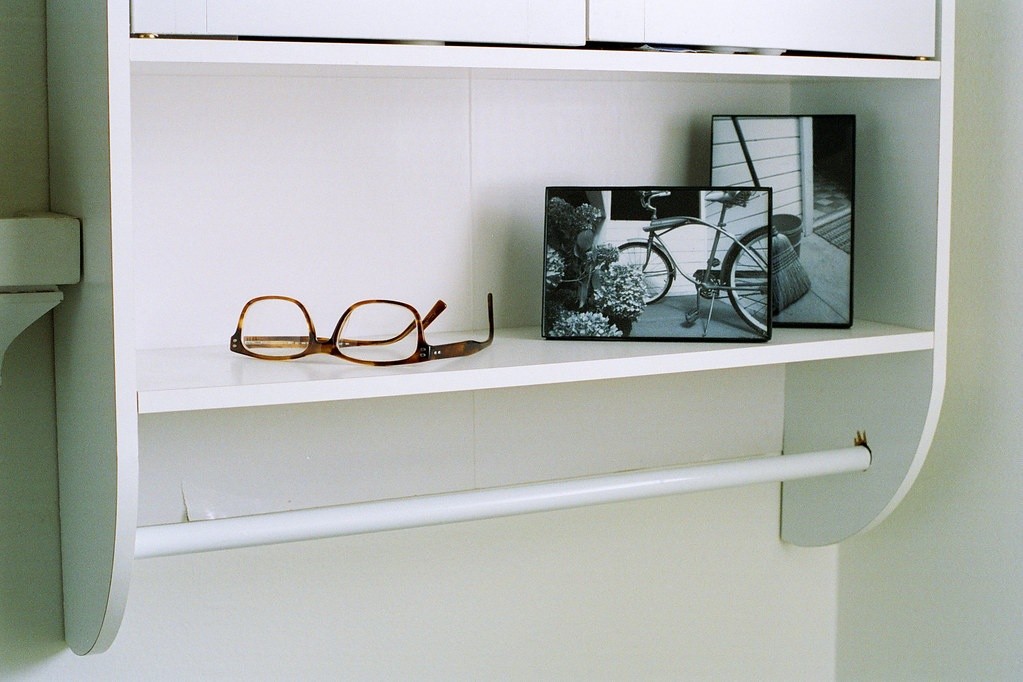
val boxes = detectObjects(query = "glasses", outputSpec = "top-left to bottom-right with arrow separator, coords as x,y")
230,293 -> 494,366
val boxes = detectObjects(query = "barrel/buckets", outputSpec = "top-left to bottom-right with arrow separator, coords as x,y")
773,213 -> 802,258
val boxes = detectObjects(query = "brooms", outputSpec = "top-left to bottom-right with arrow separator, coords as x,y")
729,117 -> 812,319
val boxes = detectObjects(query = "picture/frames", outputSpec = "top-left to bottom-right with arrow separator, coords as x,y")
540,185 -> 772,342
709,114 -> 857,328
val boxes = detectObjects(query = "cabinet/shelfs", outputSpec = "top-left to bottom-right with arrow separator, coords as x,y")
46,0 -> 954,657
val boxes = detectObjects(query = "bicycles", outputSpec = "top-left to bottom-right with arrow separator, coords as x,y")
600,190 -> 769,339
